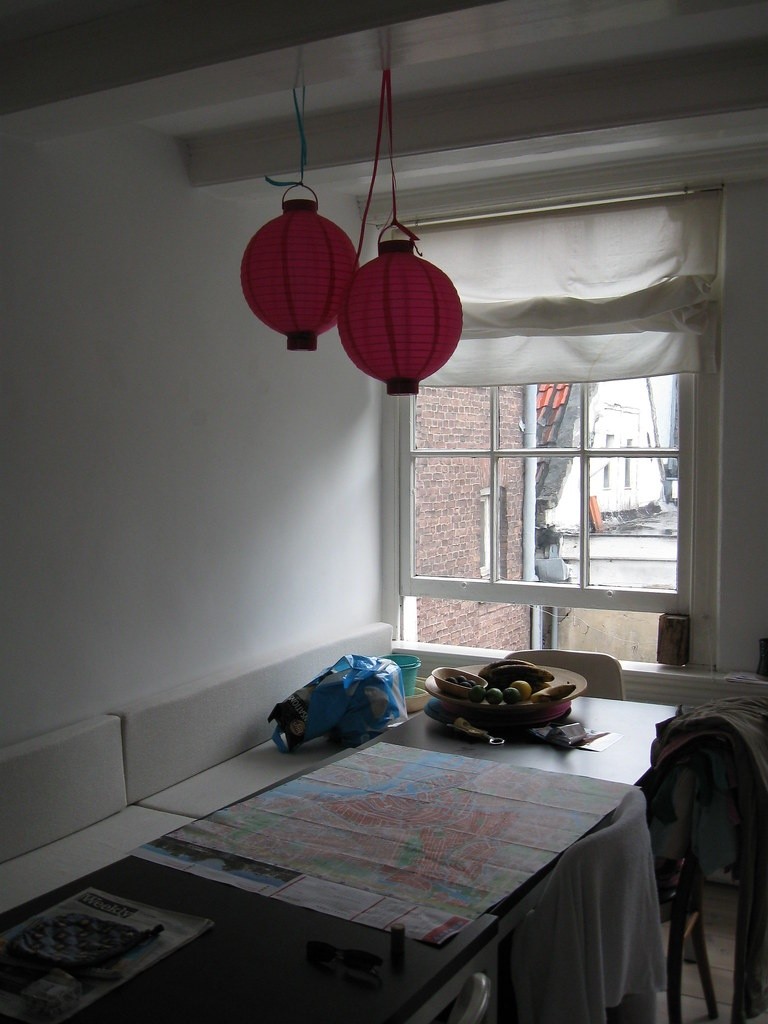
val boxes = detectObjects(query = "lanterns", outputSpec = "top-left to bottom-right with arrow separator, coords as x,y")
338,219 -> 463,396
240,185 -> 362,351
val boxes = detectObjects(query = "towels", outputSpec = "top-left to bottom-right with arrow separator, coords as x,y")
504,787 -> 667,1024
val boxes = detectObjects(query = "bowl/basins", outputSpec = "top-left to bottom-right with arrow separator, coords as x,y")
431,666 -> 489,698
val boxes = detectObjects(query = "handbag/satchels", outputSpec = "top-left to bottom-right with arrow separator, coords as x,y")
272,655 -> 409,753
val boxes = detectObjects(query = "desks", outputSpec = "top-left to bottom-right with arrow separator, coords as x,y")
168,689 -> 682,944
1,836 -> 501,1024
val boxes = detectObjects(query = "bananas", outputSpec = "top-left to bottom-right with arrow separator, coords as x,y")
478,659 -> 554,691
530,683 -> 576,703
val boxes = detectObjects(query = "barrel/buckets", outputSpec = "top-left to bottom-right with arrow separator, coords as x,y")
378,654 -> 421,695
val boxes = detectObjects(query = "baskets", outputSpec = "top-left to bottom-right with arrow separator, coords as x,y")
381,655 -> 422,696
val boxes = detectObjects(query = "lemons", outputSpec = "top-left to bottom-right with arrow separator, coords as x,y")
468,681 -> 532,705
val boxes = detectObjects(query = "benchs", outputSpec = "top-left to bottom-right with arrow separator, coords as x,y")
1,713 -> 196,916
111,621 -> 429,821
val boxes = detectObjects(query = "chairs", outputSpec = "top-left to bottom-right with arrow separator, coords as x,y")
444,972 -> 491,1024
511,790 -> 653,1023
504,650 -> 624,702
655,746 -> 720,1024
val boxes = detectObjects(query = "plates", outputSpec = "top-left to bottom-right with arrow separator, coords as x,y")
425,664 -> 588,711
